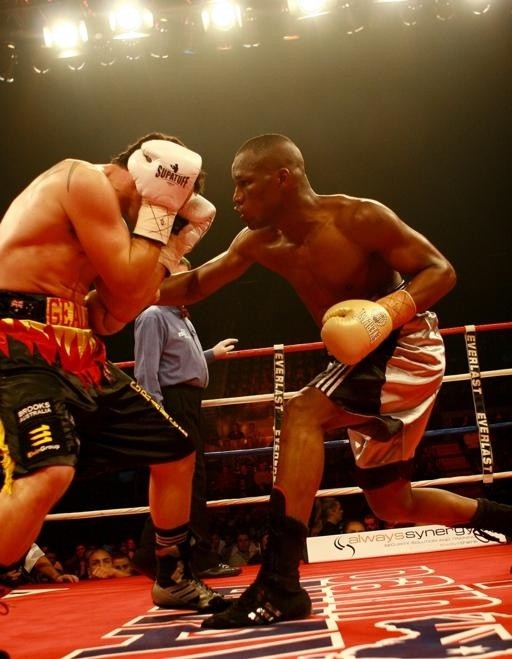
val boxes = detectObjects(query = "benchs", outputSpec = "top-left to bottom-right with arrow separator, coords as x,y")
203,415 -> 274,489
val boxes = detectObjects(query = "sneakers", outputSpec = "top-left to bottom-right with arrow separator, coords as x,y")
151,578 -> 231,612
199,585 -> 313,628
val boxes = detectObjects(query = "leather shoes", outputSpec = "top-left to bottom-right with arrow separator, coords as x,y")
193,563 -> 243,579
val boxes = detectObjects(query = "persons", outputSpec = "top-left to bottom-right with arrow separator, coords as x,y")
133,253 -> 238,612
0,131 -> 226,658
1,404 -> 511,584
82,133 -> 512,633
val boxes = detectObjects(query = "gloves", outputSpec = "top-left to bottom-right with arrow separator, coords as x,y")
158,191 -> 216,277
126,136 -> 202,246
211,337 -> 239,360
320,289 -> 420,369
